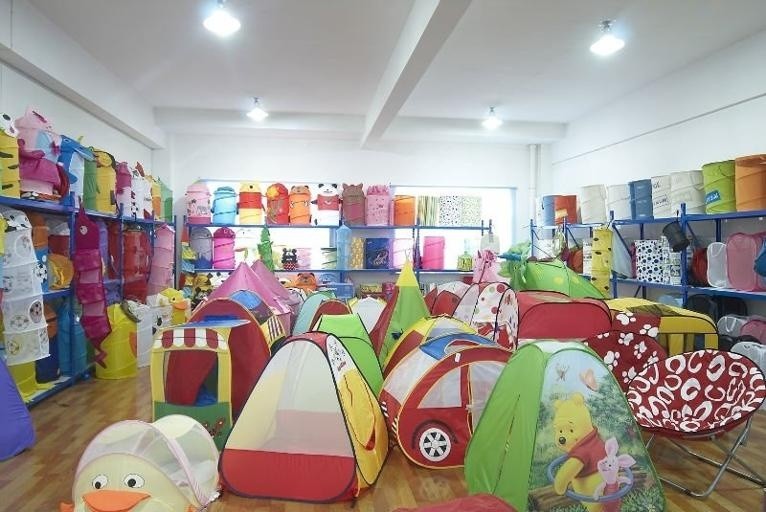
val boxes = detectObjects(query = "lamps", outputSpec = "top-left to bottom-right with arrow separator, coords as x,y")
482,107 -> 501,128
246,98 -> 268,121
202,0 -> 240,37
591,19 -> 624,54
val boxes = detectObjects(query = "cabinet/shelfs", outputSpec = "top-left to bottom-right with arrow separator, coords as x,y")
0,190 -> 178,410
530,202 -> 766,309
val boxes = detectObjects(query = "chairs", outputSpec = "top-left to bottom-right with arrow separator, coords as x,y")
625,348 -> 766,499
